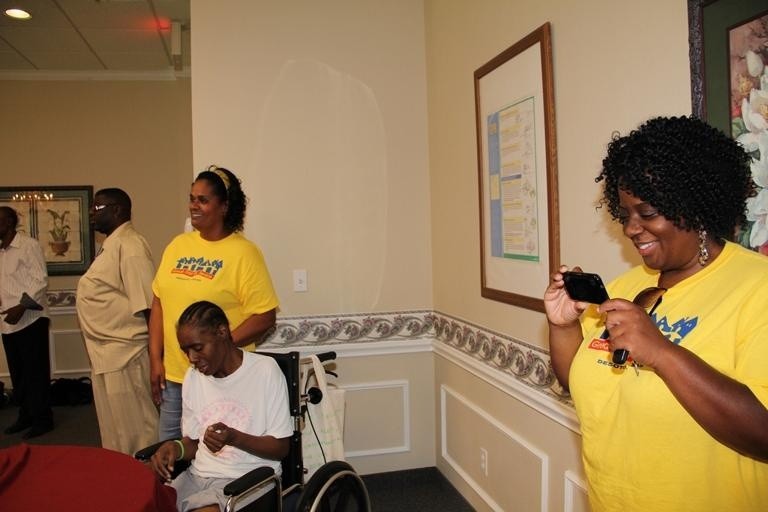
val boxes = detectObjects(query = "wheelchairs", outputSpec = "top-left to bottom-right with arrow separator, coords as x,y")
135,351 -> 371,512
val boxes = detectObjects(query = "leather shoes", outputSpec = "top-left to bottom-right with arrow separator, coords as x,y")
5,420 -> 53,438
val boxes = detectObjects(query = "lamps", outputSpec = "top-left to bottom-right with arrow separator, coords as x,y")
166,18 -> 192,62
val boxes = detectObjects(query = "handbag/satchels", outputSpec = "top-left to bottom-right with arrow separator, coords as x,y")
302,389 -> 347,485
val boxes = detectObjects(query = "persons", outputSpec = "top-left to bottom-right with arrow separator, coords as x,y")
145,162 -> 282,441
0,205 -> 57,442
73,186 -> 161,461
541,111 -> 768,511
139,297 -> 296,511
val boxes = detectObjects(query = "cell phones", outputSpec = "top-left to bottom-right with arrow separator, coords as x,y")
563,271 -> 610,305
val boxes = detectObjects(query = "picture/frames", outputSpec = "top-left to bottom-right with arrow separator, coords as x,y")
472,20 -> 563,315
686,0 -> 767,255
0,185 -> 99,277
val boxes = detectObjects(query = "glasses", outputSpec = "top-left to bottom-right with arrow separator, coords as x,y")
94,202 -> 121,212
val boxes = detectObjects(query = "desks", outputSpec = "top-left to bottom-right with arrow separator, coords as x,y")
0,443 -> 166,511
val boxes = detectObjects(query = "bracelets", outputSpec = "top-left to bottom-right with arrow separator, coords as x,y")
172,439 -> 185,462
